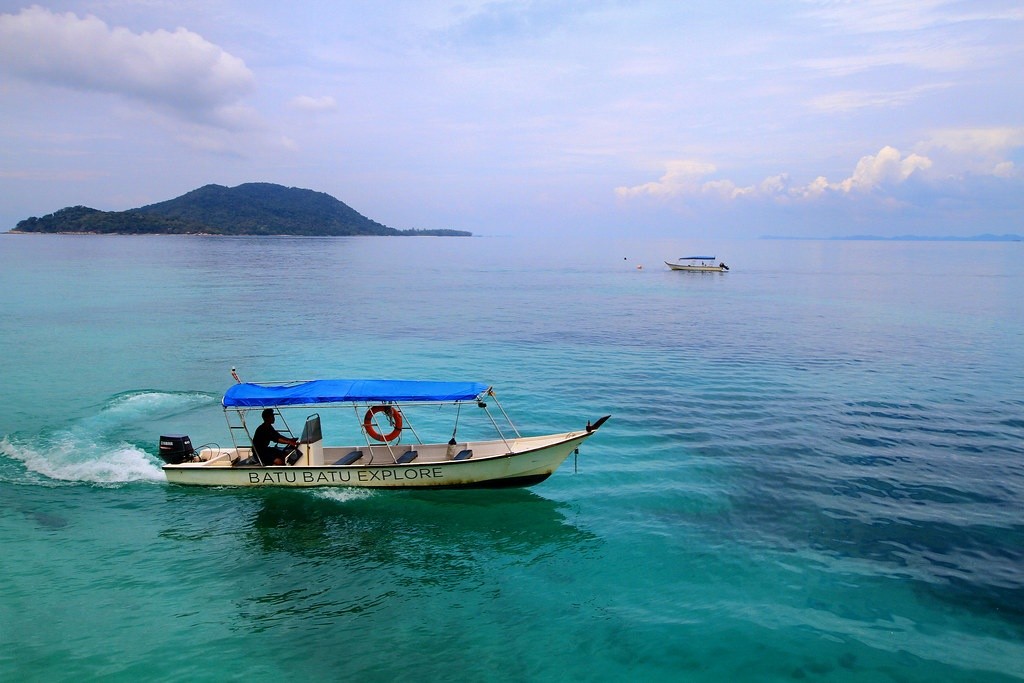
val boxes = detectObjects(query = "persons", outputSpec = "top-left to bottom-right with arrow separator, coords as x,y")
252,409 -> 297,466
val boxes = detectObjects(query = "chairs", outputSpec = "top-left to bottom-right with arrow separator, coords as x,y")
240,446 -> 279,464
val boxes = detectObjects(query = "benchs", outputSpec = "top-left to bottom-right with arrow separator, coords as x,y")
332,450 -> 364,465
395,450 -> 418,464
455,449 -> 473,460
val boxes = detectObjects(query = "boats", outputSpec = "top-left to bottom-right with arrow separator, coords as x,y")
160,366 -> 612,490
664,255 -> 729,272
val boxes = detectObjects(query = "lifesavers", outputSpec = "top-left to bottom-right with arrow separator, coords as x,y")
364,406 -> 403,441
